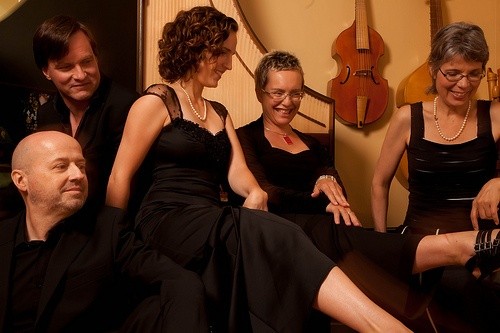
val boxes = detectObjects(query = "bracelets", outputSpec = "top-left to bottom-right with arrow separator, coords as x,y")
316,175 -> 337,184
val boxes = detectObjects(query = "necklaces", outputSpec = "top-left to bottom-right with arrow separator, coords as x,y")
433,96 -> 472,140
177,82 -> 207,121
264,125 -> 293,145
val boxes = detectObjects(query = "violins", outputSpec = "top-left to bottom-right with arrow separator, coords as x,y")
327,0 -> 389,130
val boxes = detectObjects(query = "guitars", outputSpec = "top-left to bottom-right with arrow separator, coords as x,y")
393,0 -> 445,192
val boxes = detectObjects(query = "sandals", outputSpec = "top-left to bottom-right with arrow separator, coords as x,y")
465,227 -> 500,285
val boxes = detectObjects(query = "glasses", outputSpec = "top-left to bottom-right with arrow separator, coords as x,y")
261,88 -> 304,101
439,67 -> 486,82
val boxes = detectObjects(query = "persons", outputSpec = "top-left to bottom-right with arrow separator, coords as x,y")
236,52 -> 363,227
107,7 -> 500,333
371,23 -> 500,333
24,15 -> 139,205
0,130 -> 208,333
